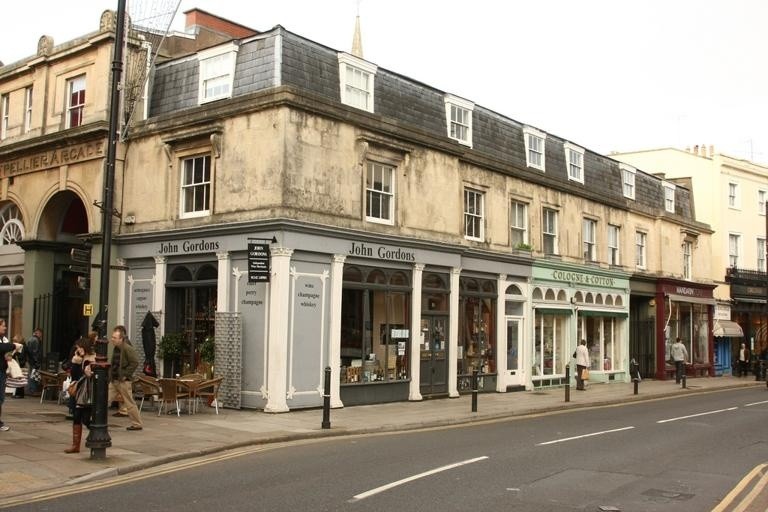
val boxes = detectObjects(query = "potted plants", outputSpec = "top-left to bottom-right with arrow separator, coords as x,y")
512,241 -> 534,257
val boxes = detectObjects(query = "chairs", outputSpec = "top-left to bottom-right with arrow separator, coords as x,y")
34,370 -> 225,418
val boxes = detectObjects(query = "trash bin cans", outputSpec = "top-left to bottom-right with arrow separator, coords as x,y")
755,360 -> 766,381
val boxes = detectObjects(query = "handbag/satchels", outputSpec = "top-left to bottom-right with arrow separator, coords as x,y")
69,382 -> 77,397
6,356 -> 29,388
581,369 -> 589,380
30,369 -> 41,382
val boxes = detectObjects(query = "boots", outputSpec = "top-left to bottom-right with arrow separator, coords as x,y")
65,425 -> 82,453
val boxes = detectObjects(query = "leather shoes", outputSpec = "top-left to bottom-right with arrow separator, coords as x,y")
112,413 -> 128,417
11,392 -> 40,398
126,425 -> 143,430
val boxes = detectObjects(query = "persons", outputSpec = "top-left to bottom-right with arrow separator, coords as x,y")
575,339 -> 590,390
11,334 -> 27,399
25,328 -> 43,397
670,337 -> 689,384
0,318 -> 22,432
736,342 -> 750,377
61,325 -> 143,453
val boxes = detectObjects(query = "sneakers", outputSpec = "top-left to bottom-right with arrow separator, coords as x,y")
0,425 -> 11,432
